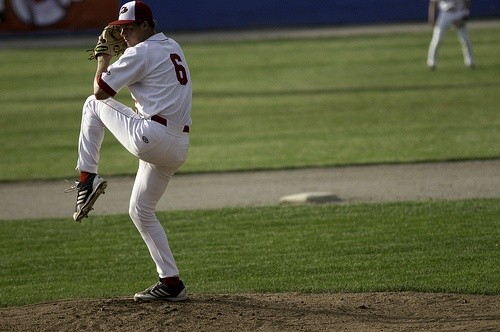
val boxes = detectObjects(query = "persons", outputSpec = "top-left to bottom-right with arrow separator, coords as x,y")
73,2 -> 192,302
427,0 -> 476,72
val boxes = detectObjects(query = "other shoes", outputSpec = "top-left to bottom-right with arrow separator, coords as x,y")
463,61 -> 475,67
427,60 -> 436,69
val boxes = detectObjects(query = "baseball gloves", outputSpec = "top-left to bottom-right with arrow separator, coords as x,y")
85,21 -> 128,61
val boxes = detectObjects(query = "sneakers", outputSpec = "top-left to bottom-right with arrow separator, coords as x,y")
133,278 -> 188,300
72,173 -> 108,221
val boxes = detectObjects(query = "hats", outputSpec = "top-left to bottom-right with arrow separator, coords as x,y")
107,0 -> 154,26
